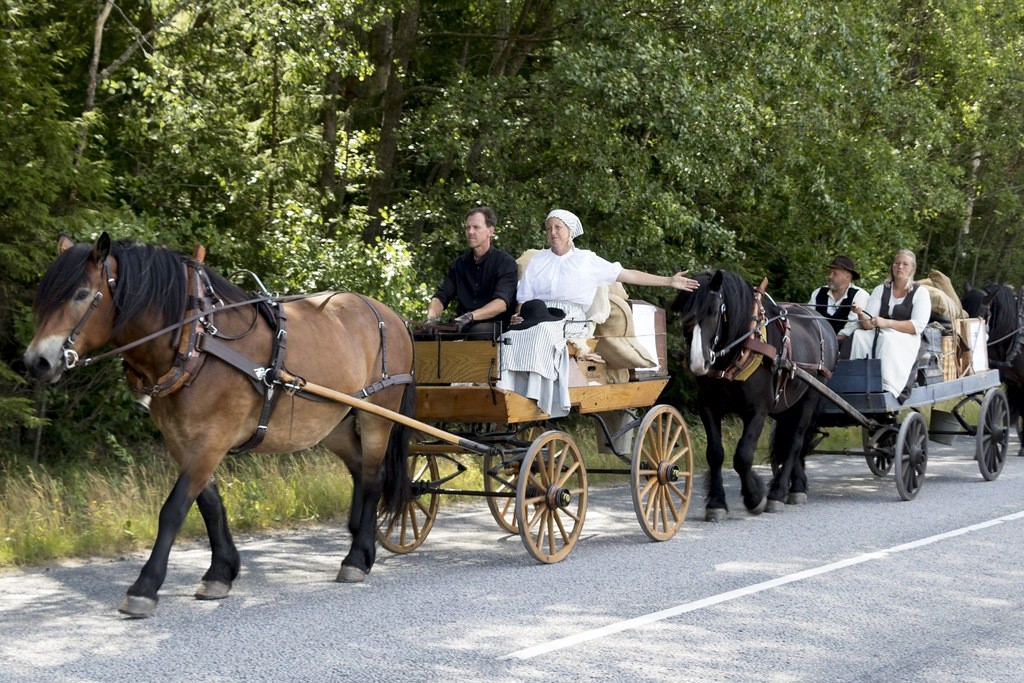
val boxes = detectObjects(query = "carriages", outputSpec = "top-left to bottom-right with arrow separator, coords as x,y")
668,265 -> 1011,525
21,229 -> 695,619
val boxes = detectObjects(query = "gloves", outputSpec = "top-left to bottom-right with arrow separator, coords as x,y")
449,312 -> 474,333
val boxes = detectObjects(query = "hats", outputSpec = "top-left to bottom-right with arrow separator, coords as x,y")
509,299 -> 567,330
825,255 -> 861,280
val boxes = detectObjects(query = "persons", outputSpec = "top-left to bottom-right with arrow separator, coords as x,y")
496,210 -> 701,419
850,249 -> 932,404
806,255 -> 870,359
414,206 -> 519,342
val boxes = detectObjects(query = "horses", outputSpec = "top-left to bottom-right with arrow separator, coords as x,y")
960,281 -> 1024,457
22,234 -> 418,619
680,269 -> 839,523
